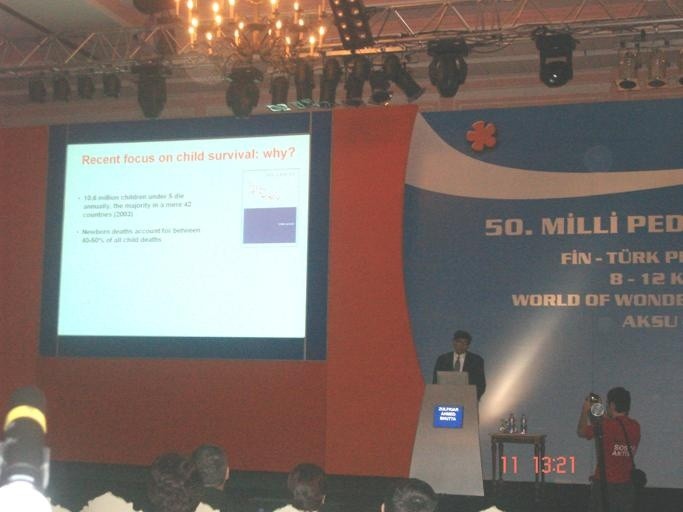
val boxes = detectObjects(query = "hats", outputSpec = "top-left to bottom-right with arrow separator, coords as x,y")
453,330 -> 472,341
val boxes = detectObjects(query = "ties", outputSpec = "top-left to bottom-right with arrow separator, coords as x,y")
455,353 -> 461,373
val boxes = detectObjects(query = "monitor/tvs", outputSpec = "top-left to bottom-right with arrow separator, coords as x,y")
436,370 -> 469,385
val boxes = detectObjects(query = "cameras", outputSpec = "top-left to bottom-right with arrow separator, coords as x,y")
589,393 -> 600,403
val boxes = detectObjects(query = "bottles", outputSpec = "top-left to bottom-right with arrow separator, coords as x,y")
508,412 -> 527,436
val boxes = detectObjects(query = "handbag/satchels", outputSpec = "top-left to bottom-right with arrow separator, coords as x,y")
631,469 -> 647,492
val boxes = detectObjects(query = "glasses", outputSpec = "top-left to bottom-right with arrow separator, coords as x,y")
455,341 -> 470,346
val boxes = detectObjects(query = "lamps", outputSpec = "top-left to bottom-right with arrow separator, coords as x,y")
131,0 -> 466,121
532,26 -> 581,88
25,66 -> 126,104
616,44 -> 681,93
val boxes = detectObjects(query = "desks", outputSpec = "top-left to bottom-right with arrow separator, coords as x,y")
490,434 -> 547,490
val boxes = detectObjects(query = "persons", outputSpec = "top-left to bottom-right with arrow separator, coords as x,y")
577,388 -> 640,512
433,331 -> 485,401
